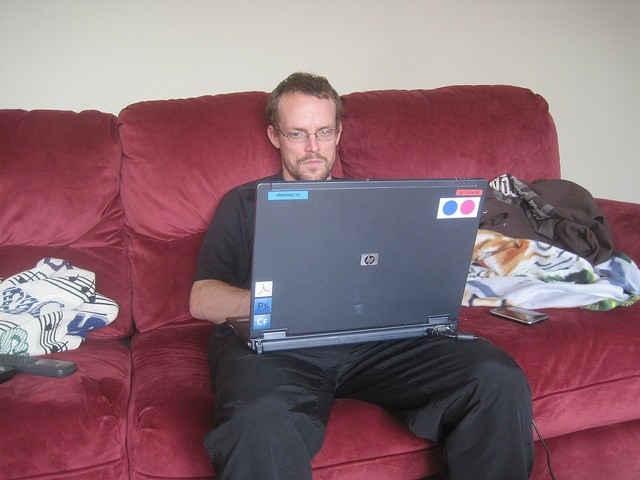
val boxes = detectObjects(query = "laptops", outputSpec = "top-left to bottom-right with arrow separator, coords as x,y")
226,177 -> 489,354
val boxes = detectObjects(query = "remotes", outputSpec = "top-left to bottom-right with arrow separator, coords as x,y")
0,354 -> 76,378
0,365 -> 16,383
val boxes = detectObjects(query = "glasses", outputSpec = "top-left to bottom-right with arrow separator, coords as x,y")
276,127 -> 339,143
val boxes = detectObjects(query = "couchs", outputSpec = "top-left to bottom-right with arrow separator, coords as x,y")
1,83 -> 640,479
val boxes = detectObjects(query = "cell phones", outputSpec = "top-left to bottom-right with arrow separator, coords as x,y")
489,304 -> 548,324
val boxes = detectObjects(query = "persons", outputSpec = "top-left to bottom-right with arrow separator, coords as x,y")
189,72 -> 534,480
